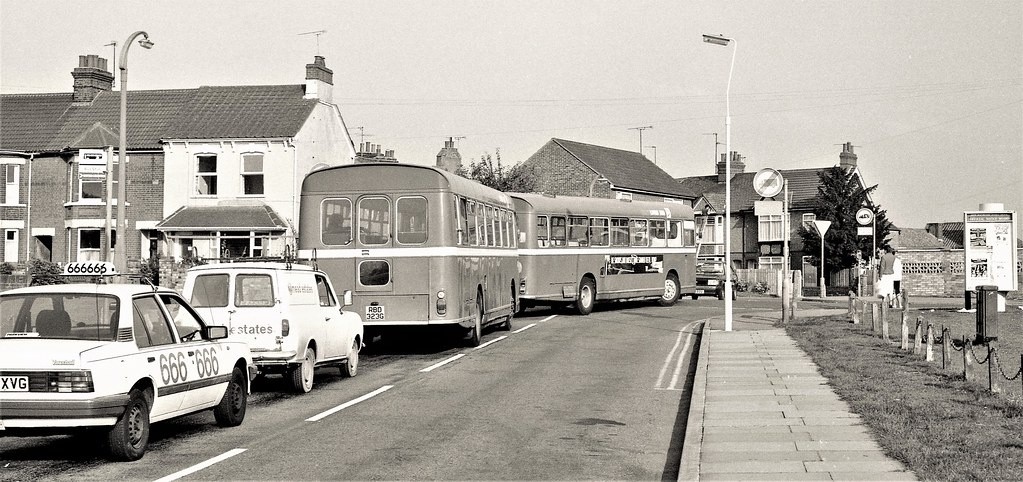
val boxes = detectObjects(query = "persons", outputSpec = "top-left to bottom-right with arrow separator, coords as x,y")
879,245 -> 903,308
699,204 -> 709,231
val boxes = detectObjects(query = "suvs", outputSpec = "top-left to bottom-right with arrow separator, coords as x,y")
173,244 -> 364,394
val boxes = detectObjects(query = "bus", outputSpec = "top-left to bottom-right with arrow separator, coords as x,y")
294,162 -> 518,348
506,190 -> 696,316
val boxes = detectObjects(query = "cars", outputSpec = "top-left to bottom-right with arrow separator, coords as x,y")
0,262 -> 258,462
691,260 -> 737,301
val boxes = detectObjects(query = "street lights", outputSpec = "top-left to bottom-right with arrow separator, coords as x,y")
703,33 -> 738,331
115,30 -> 155,285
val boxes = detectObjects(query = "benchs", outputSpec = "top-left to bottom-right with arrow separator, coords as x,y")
323,229 -> 389,245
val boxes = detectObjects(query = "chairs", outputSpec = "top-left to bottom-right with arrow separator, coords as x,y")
109,309 -> 152,347
35,310 -> 70,335
